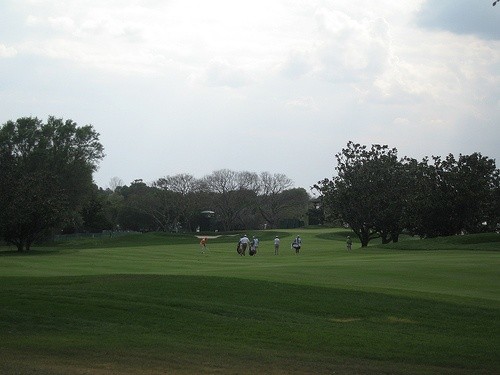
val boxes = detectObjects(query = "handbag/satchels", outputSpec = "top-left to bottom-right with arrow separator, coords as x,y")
293,243 -> 300,249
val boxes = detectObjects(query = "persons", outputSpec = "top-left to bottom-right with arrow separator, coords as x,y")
199,237 -> 207,255
273,235 -> 280,255
294,234 -> 301,253
345,236 -> 352,251
237,235 -> 259,256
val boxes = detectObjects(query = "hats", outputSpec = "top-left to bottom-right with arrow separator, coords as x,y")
244,234 -> 246,236
276,235 -> 278,237
